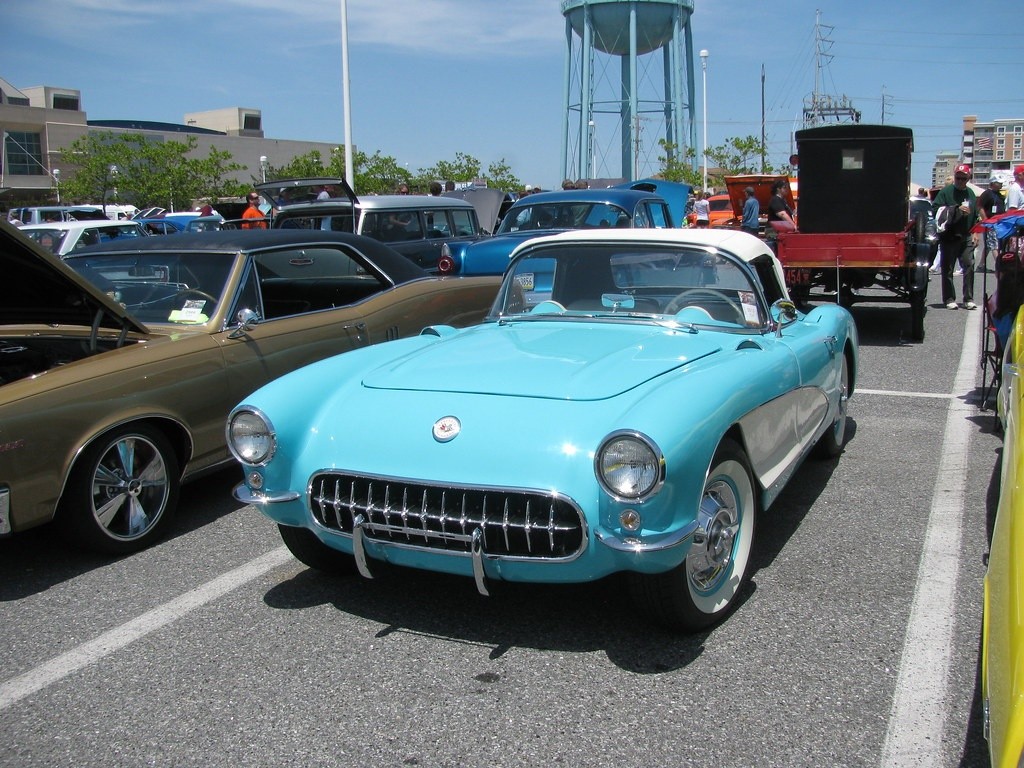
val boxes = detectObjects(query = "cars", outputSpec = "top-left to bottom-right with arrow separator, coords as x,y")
705,173 -> 798,232
227,228 -> 859,632
0,214 -> 523,560
100,218 -> 196,240
439,180 -> 692,299
13,220 -> 165,279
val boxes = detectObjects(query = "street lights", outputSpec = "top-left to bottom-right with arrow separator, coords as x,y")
699,50 -> 710,189
53,168 -> 60,202
260,155 -> 268,184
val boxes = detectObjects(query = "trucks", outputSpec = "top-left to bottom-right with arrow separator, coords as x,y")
774,124 -> 932,334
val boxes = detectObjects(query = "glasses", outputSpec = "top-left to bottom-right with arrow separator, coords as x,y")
955,176 -> 968,181
783,185 -> 786,188
400,190 -> 409,195
252,197 -> 259,200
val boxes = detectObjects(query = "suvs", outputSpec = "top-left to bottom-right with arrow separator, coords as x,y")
256,175 -> 504,277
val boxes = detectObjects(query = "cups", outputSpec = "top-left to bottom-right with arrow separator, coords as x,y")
961,201 -> 969,208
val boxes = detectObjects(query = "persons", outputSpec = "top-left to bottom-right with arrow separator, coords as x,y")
317,184 -> 335,200
910,163 -> 1024,349
741,186 -> 759,238
444,181 -> 455,193
9,212 -> 24,229
694,193 -> 710,228
510,191 -> 531,231
426,183 -> 442,197
562,178 -> 591,228
241,192 -> 269,230
121,213 -> 133,220
388,183 -> 415,233
764,179 -> 794,249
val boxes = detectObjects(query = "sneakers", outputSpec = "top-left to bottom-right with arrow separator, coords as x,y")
962,302 -> 977,309
945,303 -> 958,310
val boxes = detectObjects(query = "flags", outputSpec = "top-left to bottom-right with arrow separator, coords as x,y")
978,137 -> 993,152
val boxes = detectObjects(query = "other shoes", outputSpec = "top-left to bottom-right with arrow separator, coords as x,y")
928,270 -> 941,274
976,265 -> 992,273
953,272 -> 964,276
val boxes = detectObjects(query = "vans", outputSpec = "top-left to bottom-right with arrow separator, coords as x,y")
74,205 -> 141,221
8,207 -> 110,225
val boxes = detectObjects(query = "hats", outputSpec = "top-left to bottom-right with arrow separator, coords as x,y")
534,186 -> 541,189
989,175 -> 1005,184
954,164 -> 971,175
742,187 -> 754,193
772,179 -> 788,188
202,205 -> 212,212
1014,165 -> 1024,174
575,179 -> 588,189
526,185 -> 531,190
562,179 -> 572,187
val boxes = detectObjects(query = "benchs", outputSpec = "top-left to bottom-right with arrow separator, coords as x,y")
565,298 -> 660,314
260,279 -> 385,318
676,300 -> 744,324
